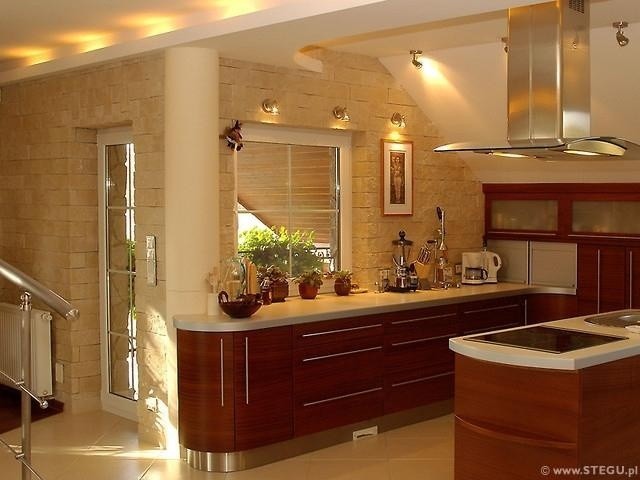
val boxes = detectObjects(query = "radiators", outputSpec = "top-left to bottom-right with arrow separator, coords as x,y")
0,301 -> 52,399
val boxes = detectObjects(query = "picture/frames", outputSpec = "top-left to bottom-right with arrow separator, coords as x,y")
380,139 -> 414,215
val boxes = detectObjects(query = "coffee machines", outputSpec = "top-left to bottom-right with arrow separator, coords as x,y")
461,252 -> 488,285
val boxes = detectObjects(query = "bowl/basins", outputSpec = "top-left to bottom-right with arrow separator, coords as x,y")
219,301 -> 262,318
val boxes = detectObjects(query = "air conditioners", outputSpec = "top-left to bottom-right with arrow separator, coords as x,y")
333,105 -> 351,124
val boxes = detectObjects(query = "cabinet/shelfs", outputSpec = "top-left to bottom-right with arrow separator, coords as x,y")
172,298 -> 292,474
568,237 -> 626,319
290,290 -> 387,458
387,280 -> 462,429
463,279 -> 536,335
481,233 -> 568,325
626,237 -> 640,309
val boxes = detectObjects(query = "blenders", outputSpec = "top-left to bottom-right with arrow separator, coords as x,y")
389,230 -> 418,292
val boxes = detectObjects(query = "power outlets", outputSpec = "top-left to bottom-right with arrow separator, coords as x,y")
55,363 -> 64,384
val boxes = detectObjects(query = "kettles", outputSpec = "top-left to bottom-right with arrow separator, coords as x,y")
483,252 -> 502,284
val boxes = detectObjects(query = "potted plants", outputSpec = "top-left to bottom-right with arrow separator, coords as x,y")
330,270 -> 354,296
256,263 -> 289,303
291,270 -> 323,299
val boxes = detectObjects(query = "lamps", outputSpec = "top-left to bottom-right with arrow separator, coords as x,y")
262,98 -> 282,117
390,111 -> 409,129
408,48 -> 426,72
611,20 -> 632,47
